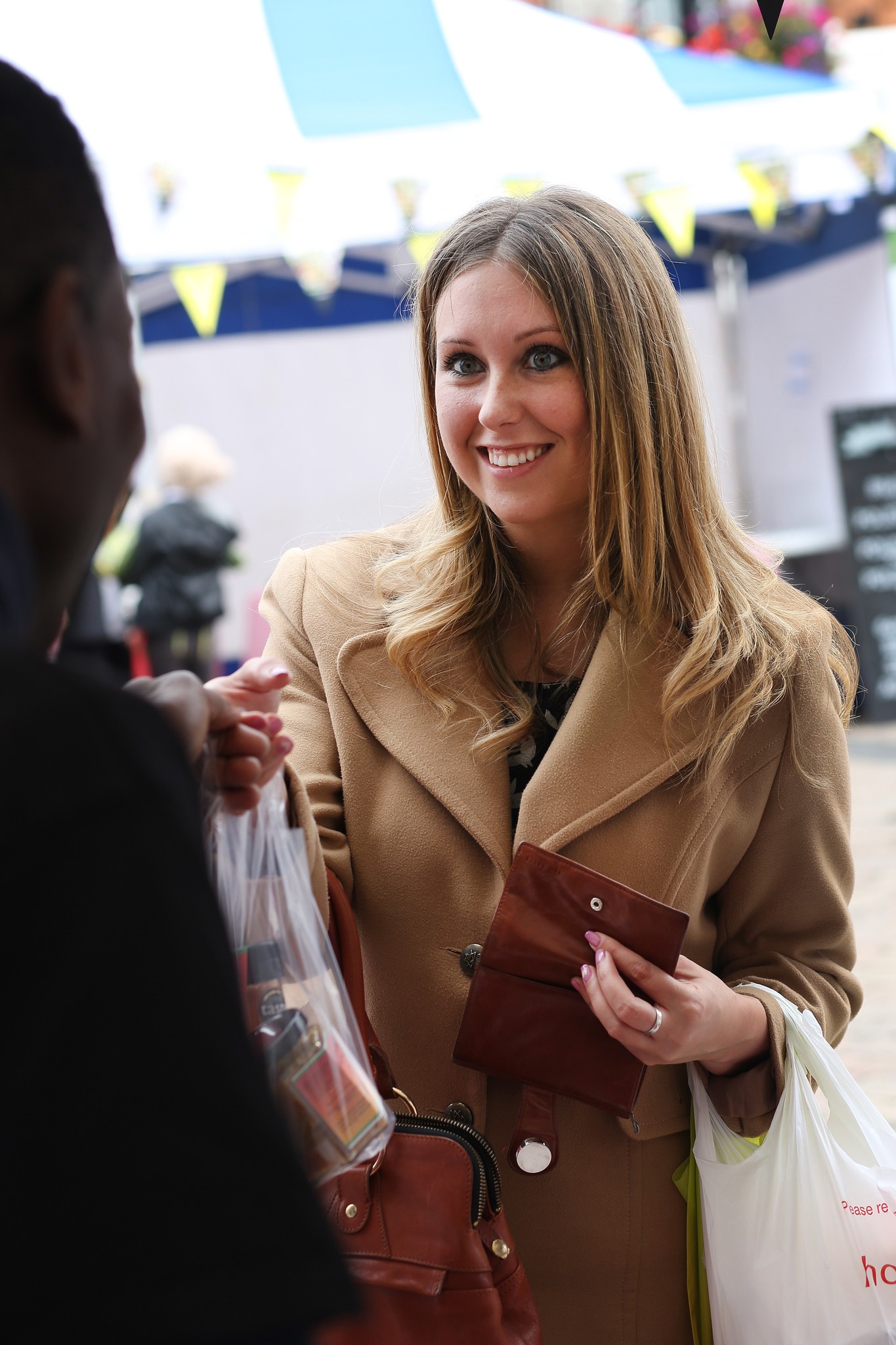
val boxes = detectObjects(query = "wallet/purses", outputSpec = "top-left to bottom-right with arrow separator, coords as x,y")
450,843 -> 687,1122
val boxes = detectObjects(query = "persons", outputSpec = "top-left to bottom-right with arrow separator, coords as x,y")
0,52 -> 382,1345
115,420 -> 240,682
200,172 -> 868,1345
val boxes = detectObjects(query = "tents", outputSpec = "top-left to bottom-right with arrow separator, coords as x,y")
0,0 -> 896,606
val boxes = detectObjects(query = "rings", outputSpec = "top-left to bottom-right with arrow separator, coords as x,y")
643,1006 -> 664,1036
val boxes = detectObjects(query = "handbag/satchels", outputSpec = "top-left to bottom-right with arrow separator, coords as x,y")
675,973 -> 896,1345
298,866 -> 540,1344
206,755 -> 397,1192
